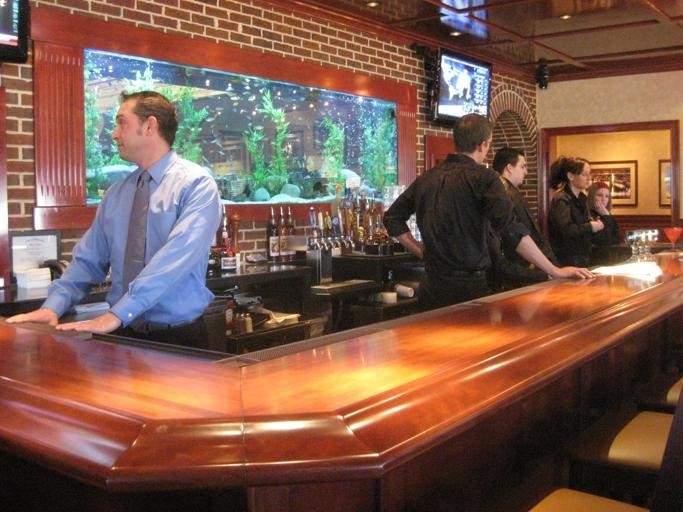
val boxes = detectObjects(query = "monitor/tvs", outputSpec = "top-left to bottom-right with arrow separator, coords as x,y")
431,47 -> 493,128
0,0 -> 30,64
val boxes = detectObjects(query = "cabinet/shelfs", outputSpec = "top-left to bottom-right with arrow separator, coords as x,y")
0,261 -> 314,349
331,239 -> 425,333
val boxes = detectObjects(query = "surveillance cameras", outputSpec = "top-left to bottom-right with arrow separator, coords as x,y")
536,57 -> 550,89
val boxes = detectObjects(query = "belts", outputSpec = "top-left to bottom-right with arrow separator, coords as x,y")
425,265 -> 487,279
146,320 -> 197,330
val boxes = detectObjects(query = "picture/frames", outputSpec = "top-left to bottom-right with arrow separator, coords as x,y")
582,160 -> 638,207
659,159 -> 673,208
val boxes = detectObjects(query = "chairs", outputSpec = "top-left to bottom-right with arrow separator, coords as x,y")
666,377 -> 683,405
568,403 -> 675,490
482,387 -> 683,512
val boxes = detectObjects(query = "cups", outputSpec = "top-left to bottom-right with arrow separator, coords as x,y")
659,253 -> 683,279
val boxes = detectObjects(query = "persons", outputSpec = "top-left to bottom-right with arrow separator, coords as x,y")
5,90 -> 223,350
586,181 -> 619,246
547,154 -> 605,266
492,147 -> 550,282
384,113 -> 598,306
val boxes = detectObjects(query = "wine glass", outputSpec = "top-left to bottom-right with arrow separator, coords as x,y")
661,227 -> 682,253
625,228 -> 658,263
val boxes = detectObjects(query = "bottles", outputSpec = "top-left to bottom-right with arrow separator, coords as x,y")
245,313 -> 254,334
233,312 -> 240,335
267,206 -> 280,265
308,184 -> 392,247
276,204 -> 288,262
285,206 -> 297,262
239,314 -> 246,337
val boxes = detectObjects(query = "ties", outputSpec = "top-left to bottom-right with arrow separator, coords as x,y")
123,170 -> 150,333
